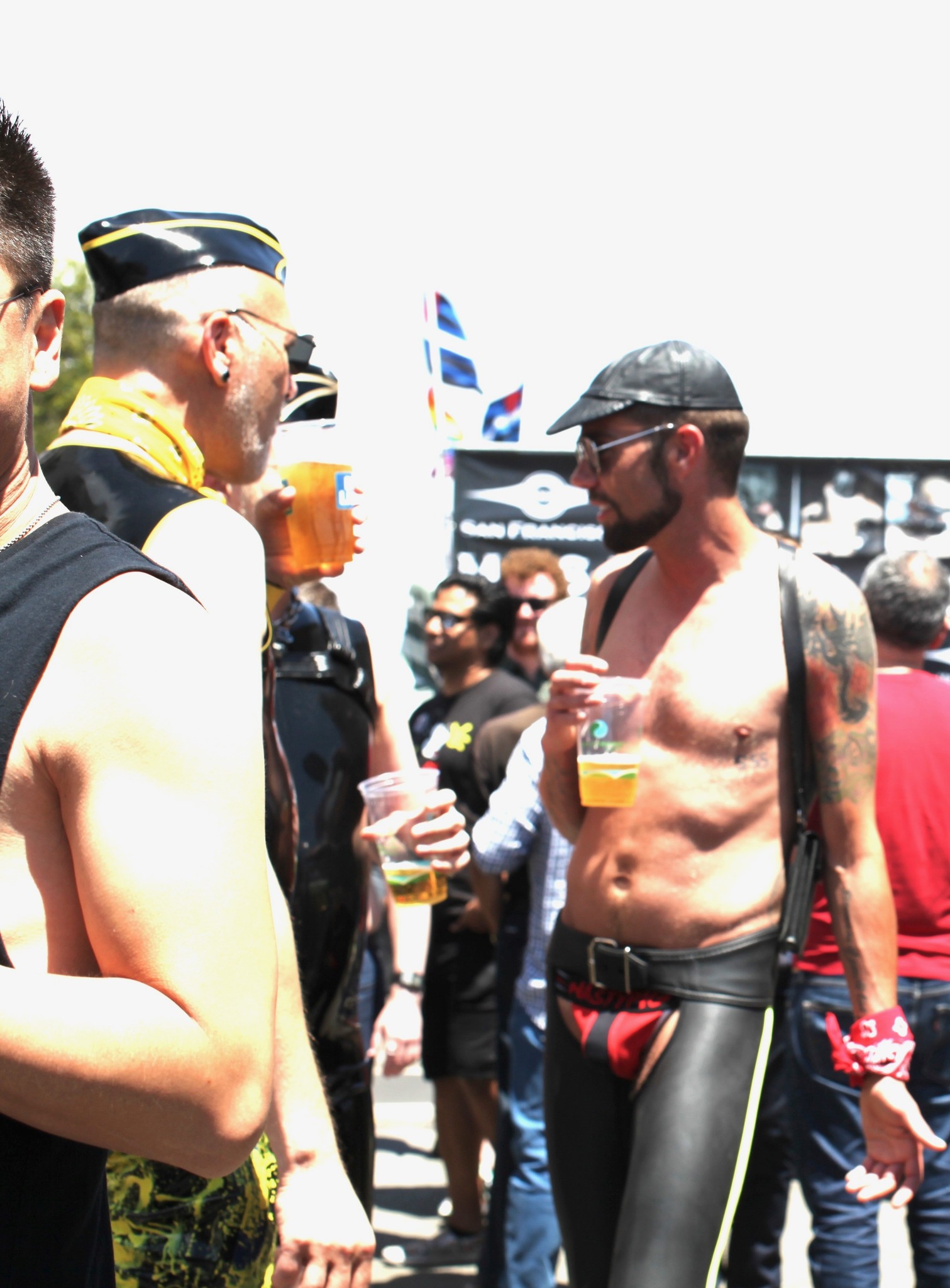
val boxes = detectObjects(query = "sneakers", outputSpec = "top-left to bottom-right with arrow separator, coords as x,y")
382,1224 -> 487,1265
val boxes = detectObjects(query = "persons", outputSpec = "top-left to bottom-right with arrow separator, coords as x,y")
406,550 -> 950,1288
0,101 -> 473,1288
540,342 -> 950,1288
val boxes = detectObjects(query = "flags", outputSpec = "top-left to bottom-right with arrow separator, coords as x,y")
423,288 -> 481,395
482,386 -> 524,441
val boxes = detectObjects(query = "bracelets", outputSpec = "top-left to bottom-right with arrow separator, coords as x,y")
825,1006 -> 915,1083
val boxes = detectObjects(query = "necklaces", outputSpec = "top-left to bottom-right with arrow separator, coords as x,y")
0,496 -> 61,552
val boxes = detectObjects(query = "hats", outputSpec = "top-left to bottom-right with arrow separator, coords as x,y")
78,209 -> 287,303
280,362 -> 338,422
545,340 -> 742,436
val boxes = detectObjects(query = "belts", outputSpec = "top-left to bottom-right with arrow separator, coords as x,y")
550,908 -> 780,1006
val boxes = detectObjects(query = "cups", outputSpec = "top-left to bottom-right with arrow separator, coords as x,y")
573,677 -> 657,810
277,437 -> 354,570
357,768 -> 448,905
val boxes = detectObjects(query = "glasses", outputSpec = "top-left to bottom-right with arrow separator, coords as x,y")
227,308 -> 316,373
504,598 -> 556,611
423,607 -> 471,628
576,423 -> 677,474
